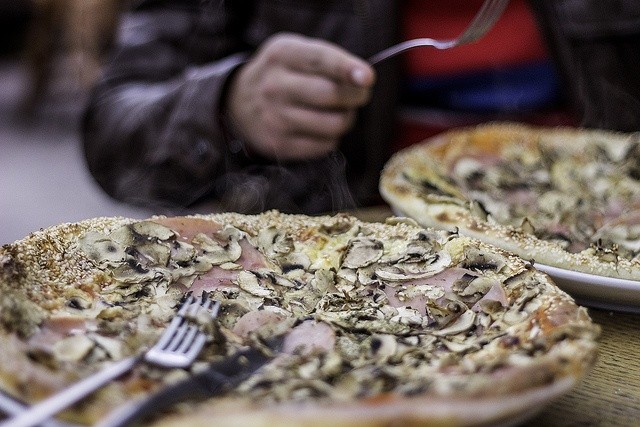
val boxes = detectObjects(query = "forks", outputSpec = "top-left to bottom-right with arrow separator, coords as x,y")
365,1 -> 510,69
1,295 -> 223,426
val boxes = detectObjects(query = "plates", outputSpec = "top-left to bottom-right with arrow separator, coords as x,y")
390,203 -> 639,313
1,351 -> 602,426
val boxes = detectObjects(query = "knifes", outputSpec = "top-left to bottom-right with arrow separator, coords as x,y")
92,333 -> 285,426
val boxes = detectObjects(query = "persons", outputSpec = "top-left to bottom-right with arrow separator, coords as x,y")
82,0 -> 640,214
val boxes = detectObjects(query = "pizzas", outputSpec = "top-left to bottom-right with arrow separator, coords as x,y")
380,121 -> 635,279
2,211 -> 600,427
